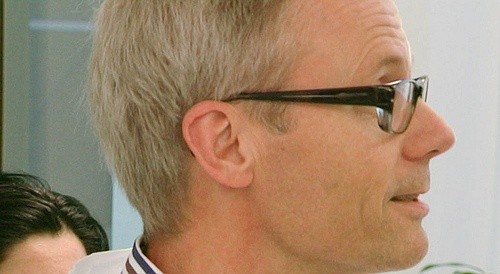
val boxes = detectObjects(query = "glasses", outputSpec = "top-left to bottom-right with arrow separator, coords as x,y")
221,75 -> 429,134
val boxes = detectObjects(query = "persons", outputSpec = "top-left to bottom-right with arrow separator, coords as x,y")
0,171 -> 109,274
63,0 -> 452,274
